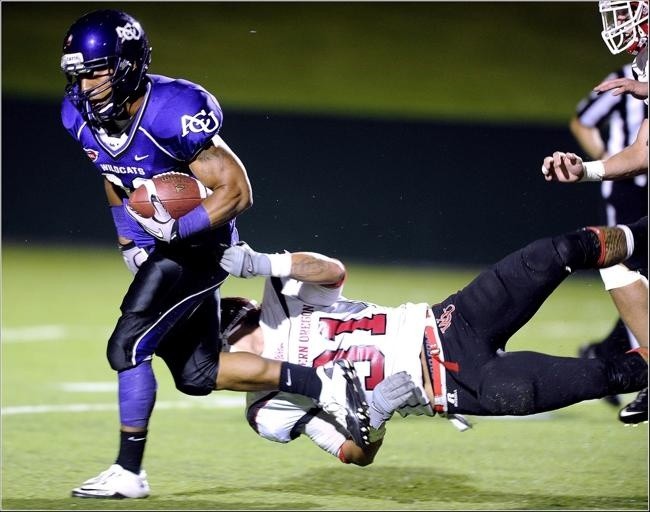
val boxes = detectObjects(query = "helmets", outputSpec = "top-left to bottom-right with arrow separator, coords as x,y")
597,0 -> 650,56
58,6 -> 156,130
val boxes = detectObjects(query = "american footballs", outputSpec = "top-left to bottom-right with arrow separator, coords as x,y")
124,170 -> 218,223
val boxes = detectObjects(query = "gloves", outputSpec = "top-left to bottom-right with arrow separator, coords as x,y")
366,369 -> 421,431
117,194 -> 180,244
218,239 -> 269,284
116,232 -> 152,278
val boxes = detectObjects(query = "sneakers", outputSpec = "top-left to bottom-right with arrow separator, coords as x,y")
314,356 -> 375,454
616,380 -> 647,423
67,458 -> 155,503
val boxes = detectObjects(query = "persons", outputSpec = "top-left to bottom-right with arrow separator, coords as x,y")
541,1 -> 650,429
56,9 -> 372,501
217,216 -> 650,468
567,62 -> 648,409
